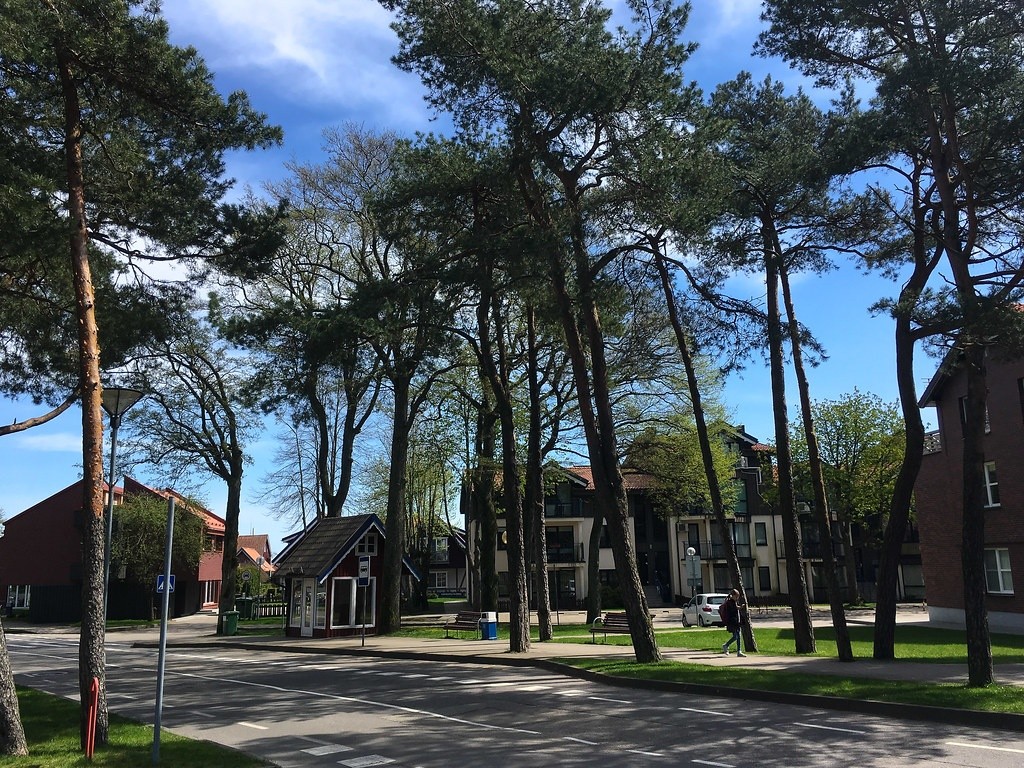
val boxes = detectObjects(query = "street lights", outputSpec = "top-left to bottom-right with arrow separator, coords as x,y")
256,555 -> 265,620
686,545 -> 701,628
99,370 -> 151,620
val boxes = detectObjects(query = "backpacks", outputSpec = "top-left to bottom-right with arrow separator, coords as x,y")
719,602 -> 729,625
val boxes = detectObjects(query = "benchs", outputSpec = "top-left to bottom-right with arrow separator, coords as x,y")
589,613 -> 656,643
443,611 -> 498,639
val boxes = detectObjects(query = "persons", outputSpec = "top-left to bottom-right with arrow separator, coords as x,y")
722,588 -> 747,657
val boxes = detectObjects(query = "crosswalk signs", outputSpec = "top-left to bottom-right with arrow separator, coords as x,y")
157,575 -> 176,593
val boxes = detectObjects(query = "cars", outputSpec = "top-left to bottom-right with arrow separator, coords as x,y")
295,592 -> 326,603
681,592 -> 729,628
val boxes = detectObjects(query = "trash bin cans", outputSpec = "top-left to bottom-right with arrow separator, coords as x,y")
222,611 -> 240,636
481,611 -> 498,640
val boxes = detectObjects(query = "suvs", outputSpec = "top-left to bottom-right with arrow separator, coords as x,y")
253,588 -> 285,601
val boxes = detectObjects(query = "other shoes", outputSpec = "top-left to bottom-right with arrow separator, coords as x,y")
737,652 -> 746,657
722,644 -> 729,655
